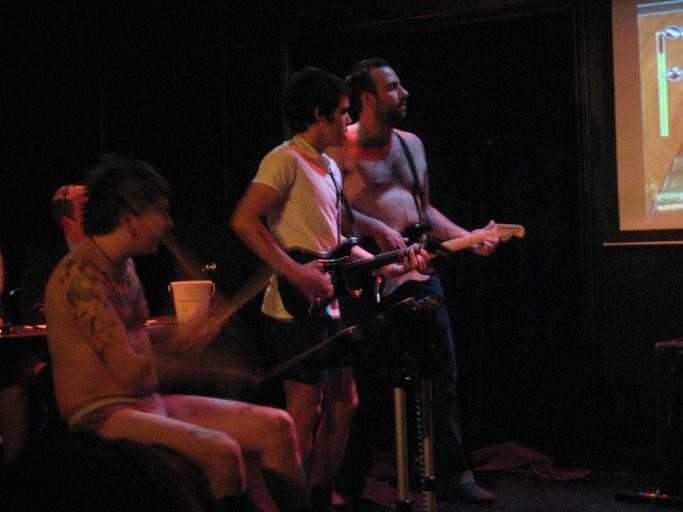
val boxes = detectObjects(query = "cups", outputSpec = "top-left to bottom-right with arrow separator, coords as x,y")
170,279 -> 215,324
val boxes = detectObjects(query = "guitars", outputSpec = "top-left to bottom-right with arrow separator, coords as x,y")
275,236 -> 453,336
343,221 -> 525,297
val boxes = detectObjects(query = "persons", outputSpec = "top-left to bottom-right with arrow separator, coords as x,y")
0,255 -> 28,463
322,58 -> 506,509
20,181 -> 93,418
223,59 -> 439,510
44,153 -> 315,509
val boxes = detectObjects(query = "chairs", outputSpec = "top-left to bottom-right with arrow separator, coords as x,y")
15,358 -> 172,510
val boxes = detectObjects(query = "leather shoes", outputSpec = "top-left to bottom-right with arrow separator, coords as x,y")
436,470 -> 498,507
